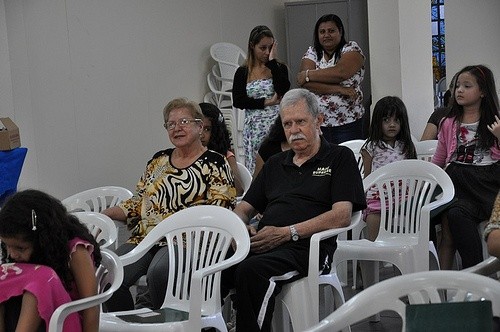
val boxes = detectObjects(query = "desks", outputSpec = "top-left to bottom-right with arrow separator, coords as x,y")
0,148 -> 27,205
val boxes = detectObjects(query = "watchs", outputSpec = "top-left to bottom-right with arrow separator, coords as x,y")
306,70 -> 309,82
289,224 -> 299,242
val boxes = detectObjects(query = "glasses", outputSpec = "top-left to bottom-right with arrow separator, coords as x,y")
164,119 -> 202,129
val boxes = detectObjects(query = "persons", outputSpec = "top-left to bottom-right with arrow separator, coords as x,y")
296,14 -> 366,144
187,88 -> 367,332
420,65 -> 500,270
232,25 -> 291,174
0,189 -> 100,332
99,98 -> 236,312
360,96 -> 422,242
198,103 -> 243,197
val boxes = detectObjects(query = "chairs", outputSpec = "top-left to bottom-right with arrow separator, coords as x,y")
49,42 -> 500,332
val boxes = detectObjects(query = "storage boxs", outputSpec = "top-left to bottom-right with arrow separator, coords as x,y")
0,117 -> 21,151
